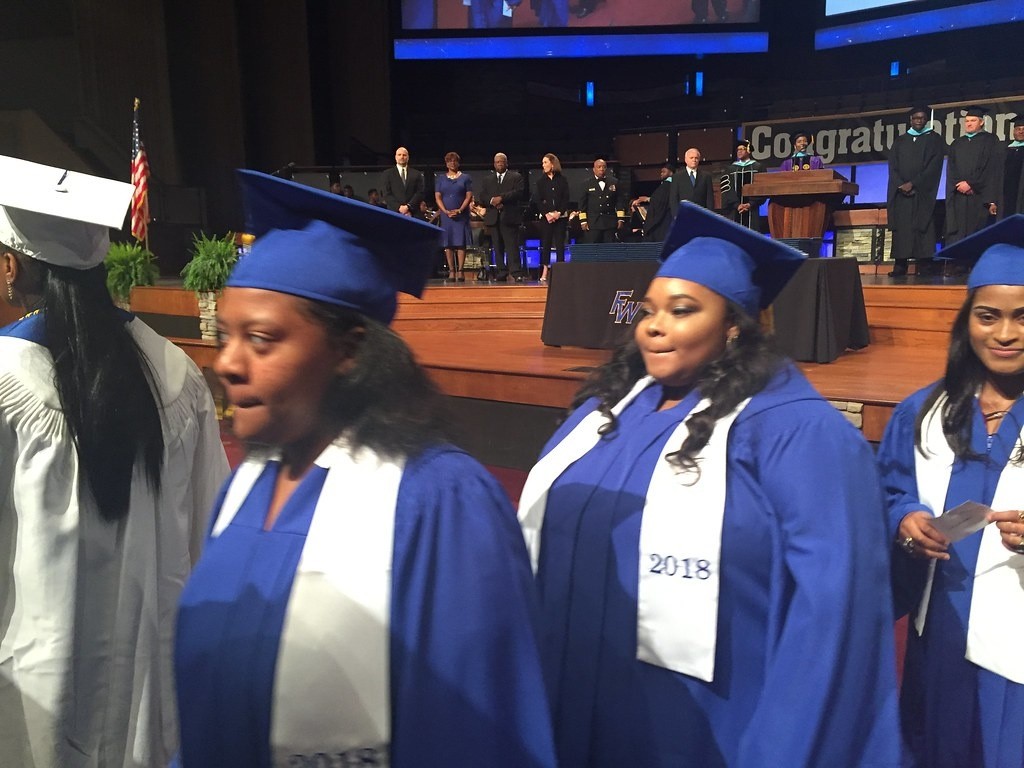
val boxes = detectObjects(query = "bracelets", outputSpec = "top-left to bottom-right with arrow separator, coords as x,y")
458,209 -> 462,214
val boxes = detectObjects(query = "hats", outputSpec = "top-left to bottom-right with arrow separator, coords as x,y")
222,168 -> 445,326
654,200 -> 809,318
935,215 -> 1024,289
788,129 -> 814,147
961,105 -> 989,131
735,139 -> 754,154
1009,114 -> 1024,128
905,105 -> 934,130
0,154 -> 136,270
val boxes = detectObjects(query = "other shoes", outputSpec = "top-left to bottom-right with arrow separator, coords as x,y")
457,269 -> 465,281
515,276 -> 522,282
446,269 -> 455,282
887,271 -> 905,276
541,277 -> 547,282
493,277 -> 507,281
917,270 -> 930,276
944,266 -> 969,277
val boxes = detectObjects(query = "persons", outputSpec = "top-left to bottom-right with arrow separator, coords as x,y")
690,0 -> 727,24
0,153 -> 233,768
670,148 -> 713,217
578,158 -> 625,243
632,163 -> 675,241
463,0 -> 598,29
720,140 -> 767,230
779,128 -> 824,170
434,152 -> 473,282
873,212 -> 1024,768
515,199 -> 916,768
887,105 -> 1024,278
479,153 -> 526,282
176,166 -> 560,768
331,181 -> 387,208
532,153 -> 569,281
380,146 -> 425,217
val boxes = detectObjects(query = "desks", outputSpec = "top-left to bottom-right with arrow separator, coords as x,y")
540,257 -> 871,365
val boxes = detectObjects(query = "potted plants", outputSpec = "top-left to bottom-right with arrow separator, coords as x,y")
103,239 -> 162,312
177,229 -> 240,341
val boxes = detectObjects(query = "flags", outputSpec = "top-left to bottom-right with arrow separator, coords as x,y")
131,107 -> 153,242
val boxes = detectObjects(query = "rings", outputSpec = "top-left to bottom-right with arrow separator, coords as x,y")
1018,511 -> 1024,522
904,537 -> 914,548
1019,539 -> 1024,546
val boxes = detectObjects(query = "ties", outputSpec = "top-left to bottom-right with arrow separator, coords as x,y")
498,175 -> 502,184
401,169 -> 406,187
690,171 -> 695,186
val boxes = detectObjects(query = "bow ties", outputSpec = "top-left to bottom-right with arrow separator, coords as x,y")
595,178 -> 607,183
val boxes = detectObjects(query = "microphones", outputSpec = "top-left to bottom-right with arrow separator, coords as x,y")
794,145 -> 806,172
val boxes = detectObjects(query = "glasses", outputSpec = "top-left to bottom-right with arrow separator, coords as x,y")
736,148 -> 747,152
